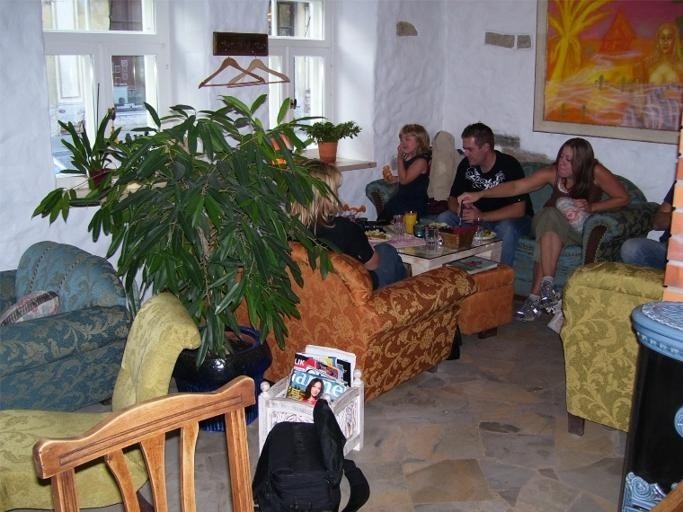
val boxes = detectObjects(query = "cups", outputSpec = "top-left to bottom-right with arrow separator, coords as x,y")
404,212 -> 418,235
393,214 -> 408,241
425,227 -> 439,252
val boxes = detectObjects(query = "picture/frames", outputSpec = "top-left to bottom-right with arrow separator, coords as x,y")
528,0 -> 682,147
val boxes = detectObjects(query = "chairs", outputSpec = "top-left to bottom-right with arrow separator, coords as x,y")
557,259 -> 666,437
202,236 -> 478,404
0,292 -> 203,511
0,238 -> 132,412
31,373 -> 257,512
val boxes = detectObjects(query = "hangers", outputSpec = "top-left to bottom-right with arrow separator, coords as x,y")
194,43 -> 264,90
229,46 -> 291,87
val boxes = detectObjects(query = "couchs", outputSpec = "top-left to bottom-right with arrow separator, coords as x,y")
364,154 -> 661,308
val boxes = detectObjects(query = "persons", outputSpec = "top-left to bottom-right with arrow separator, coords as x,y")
377,123 -> 629,322
289,158 -> 412,291
636,22 -> 683,84
619,180 -> 676,272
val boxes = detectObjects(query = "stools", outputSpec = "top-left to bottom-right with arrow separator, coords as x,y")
446,258 -> 516,343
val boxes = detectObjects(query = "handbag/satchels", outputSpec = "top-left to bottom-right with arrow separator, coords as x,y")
251,399 -> 370,512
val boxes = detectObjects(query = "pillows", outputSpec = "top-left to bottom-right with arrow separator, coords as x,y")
0,287 -> 60,328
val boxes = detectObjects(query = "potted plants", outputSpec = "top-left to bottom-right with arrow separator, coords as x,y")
31,91 -> 346,430
300,120 -> 363,160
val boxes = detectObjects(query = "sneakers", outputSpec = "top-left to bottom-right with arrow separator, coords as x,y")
511,294 -> 543,322
537,276 -> 560,310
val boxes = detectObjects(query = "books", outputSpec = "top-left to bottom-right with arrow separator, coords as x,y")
285,343 -> 357,407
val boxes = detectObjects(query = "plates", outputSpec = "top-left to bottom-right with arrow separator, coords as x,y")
474,232 -> 497,240
369,233 -> 392,242
